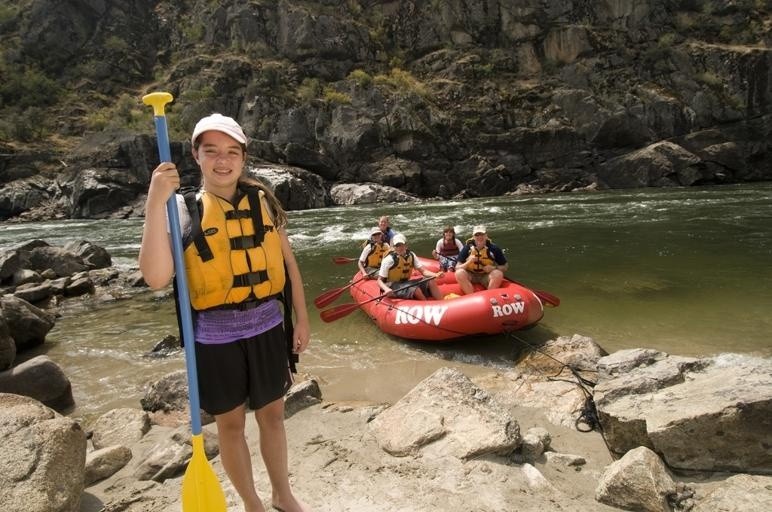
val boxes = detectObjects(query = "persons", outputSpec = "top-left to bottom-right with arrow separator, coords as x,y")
362,217 -> 395,248
358,227 -> 390,280
377,234 -> 445,300
432,227 -> 464,272
139,113 -> 310,512
455,226 -> 509,294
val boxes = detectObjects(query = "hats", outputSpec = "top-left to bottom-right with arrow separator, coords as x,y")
191,113 -> 248,146
473,226 -> 486,235
392,233 -> 407,246
370,226 -> 382,235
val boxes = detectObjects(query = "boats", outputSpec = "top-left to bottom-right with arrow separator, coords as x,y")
350,255 -> 543,341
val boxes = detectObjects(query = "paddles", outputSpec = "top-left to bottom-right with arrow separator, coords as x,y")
503,277 -> 560,308
315,270 -> 443,324
332,257 -> 360,266
140,91 -> 230,512
